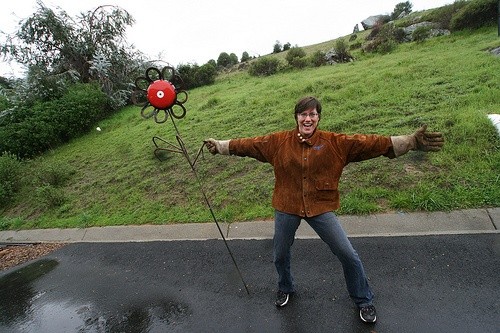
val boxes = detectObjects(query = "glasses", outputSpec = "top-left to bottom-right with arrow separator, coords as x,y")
297,113 -> 320,118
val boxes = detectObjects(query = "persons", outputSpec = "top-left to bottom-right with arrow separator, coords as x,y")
204,96 -> 445,323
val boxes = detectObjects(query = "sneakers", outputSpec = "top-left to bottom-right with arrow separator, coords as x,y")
359,305 -> 377,322
275,290 -> 289,306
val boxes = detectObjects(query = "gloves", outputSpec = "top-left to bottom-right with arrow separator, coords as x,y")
390,125 -> 445,158
203,138 -> 232,156
